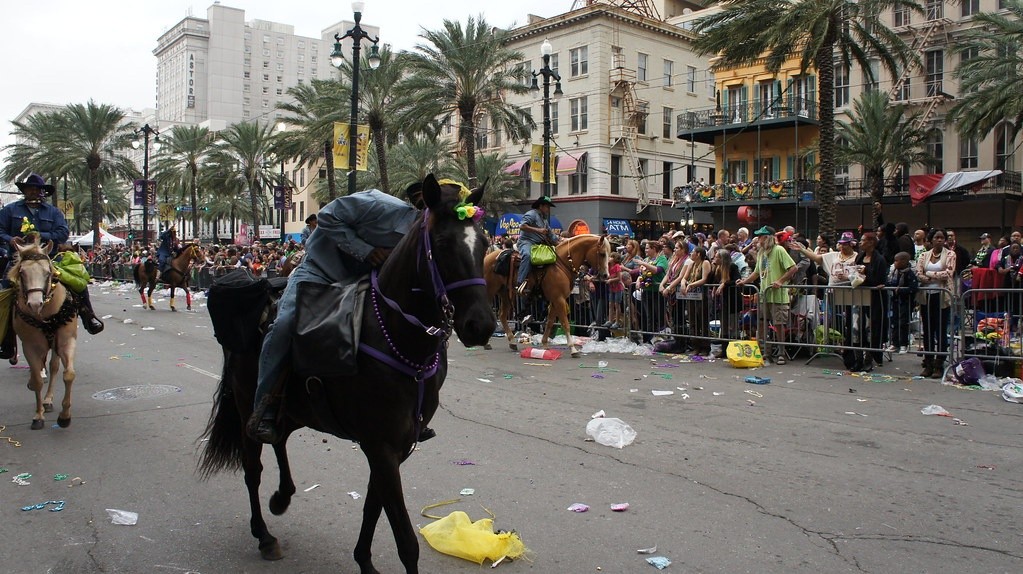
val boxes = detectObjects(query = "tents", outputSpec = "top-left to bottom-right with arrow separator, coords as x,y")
73,229 -> 125,245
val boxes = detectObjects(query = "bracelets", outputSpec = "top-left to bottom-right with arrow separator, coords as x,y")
666,286 -> 670,290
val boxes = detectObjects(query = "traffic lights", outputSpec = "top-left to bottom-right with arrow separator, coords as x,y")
128,234 -> 134,239
159,223 -> 163,234
204,206 -> 209,211
174,205 -> 180,211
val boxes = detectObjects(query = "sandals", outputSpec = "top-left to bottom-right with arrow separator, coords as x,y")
776,354 -> 787,365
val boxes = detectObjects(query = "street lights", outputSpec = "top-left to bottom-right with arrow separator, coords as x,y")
680,193 -> 694,235
530,38 -> 563,197
330,0 -> 381,194
248,228 -> 254,244
277,121 -> 286,243
132,123 -> 162,247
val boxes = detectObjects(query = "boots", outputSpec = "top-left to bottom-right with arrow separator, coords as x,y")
155,270 -> 161,282
0,308 -> 14,359
850,349 -> 863,371
861,351 -> 874,371
72,285 -> 104,335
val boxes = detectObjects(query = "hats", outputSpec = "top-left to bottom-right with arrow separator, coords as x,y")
532,196 -> 556,209
837,232 -> 857,243
305,214 -> 316,224
754,225 -> 775,236
671,230 -> 685,239
16,175 -> 55,197
979,232 -> 992,239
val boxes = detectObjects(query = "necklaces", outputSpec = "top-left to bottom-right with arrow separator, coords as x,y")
933,253 -> 941,258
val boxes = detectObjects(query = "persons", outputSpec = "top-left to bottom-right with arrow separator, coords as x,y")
194,235 -> 298,275
690,177 -> 704,187
515,196 -> 556,293
254,183 -> 462,440
568,222 -> 1023,378
156,225 -> 180,280
300,213 -> 318,245
487,233 -> 518,251
84,240 -> 161,267
0,175 -> 104,335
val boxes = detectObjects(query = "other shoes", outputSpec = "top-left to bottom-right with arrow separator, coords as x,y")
515,284 -> 531,294
412,422 -> 435,444
601,321 -> 620,329
885,344 -> 899,352
931,367 -> 944,378
899,345 -> 907,354
920,367 -> 934,377
248,409 -> 278,441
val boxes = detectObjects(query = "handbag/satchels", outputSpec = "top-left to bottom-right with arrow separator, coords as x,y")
530,243 -> 557,265
726,340 -> 764,368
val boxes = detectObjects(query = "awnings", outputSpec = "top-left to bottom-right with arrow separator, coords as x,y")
556,151 -> 588,176
505,158 -> 530,177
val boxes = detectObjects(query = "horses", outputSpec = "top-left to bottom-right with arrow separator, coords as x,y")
280,250 -> 306,277
189,171 -> 499,574
9,237 -> 81,431
132,242 -> 205,312
482,233 -> 613,359
